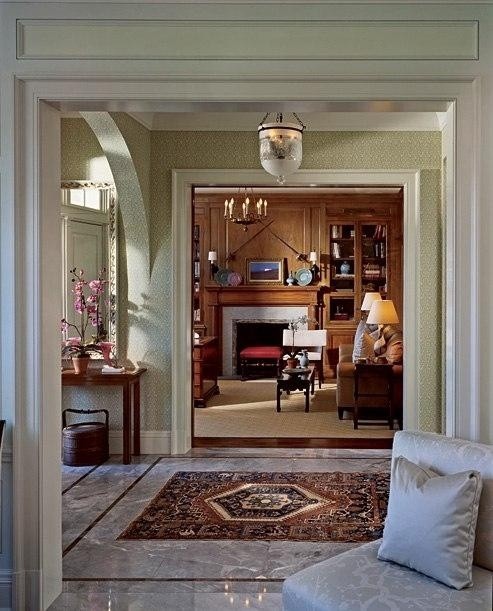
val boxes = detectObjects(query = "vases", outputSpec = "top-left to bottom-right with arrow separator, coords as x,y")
71,355 -> 89,375
298,349 -> 308,367
286,359 -> 297,369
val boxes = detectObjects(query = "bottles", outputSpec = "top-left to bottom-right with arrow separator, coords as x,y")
341,261 -> 350,273
300,349 -> 309,369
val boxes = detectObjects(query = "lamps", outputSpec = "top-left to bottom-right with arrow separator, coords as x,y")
255,111 -> 306,185
206,247 -> 220,279
222,187 -> 270,230
364,298 -> 401,365
359,291 -> 384,312
308,247 -> 318,282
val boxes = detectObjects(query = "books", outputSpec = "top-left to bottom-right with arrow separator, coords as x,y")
363,225 -> 387,278
330,225 -> 343,259
194,226 -> 201,322
331,264 -> 355,278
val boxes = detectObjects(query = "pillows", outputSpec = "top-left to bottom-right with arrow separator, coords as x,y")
350,320 -> 386,363
376,453 -> 484,588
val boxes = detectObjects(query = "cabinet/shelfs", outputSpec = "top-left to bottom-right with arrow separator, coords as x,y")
191,333 -> 221,409
193,222 -> 204,326
322,325 -> 361,385
324,218 -> 389,323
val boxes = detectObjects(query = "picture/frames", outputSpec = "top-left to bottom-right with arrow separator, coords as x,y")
244,256 -> 286,287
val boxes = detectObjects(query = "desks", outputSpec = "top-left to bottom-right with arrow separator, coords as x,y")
59,363 -> 147,465
351,362 -> 398,429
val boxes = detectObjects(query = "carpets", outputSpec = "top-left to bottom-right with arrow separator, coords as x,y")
110,470 -> 391,545
189,374 -> 404,439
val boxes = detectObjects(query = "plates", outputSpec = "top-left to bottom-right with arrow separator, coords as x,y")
296,267 -> 313,285
215,269 -> 232,285
228,272 -> 242,285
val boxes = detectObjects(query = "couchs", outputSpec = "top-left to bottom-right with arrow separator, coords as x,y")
336,323 -> 403,426
279,428 -> 492,610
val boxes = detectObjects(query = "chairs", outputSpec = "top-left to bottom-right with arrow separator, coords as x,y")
280,329 -> 327,389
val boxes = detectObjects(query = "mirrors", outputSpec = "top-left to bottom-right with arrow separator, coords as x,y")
57,181 -> 118,362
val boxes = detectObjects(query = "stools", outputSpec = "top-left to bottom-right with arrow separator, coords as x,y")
238,346 -> 284,382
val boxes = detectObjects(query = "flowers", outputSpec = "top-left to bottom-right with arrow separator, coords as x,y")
286,314 -> 320,329
86,265 -> 111,336
56,266 -> 102,356
